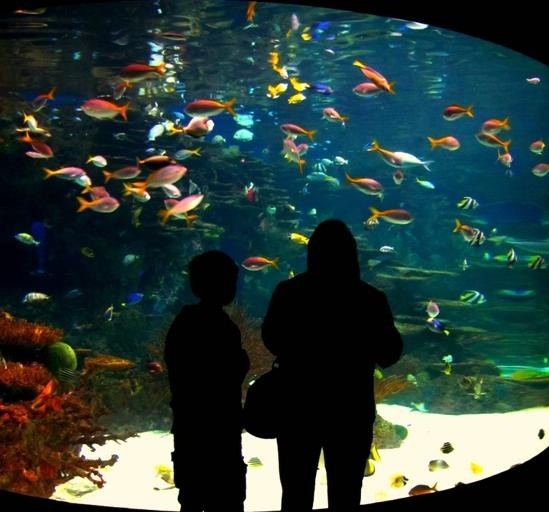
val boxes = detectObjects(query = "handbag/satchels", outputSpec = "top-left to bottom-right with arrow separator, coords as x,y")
244,363 -> 287,440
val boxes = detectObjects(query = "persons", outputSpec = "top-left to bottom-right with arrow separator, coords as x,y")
164,249 -> 250,512
262,219 -> 404,511
